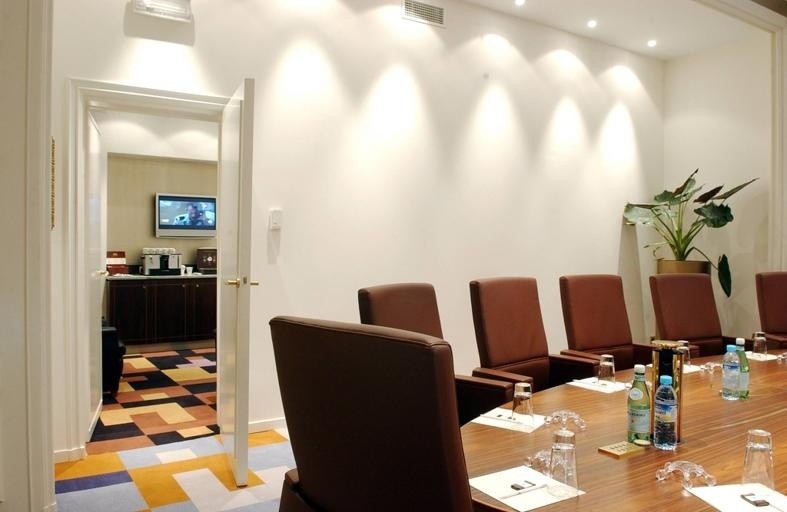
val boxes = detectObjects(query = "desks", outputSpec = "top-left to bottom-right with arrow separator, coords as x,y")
460,347 -> 787,511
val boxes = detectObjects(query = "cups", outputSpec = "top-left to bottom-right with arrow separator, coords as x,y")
509,380 -> 536,425
595,353 -> 618,388
750,330 -> 771,361
186,267 -> 194,277
739,425 -> 778,490
677,342 -> 692,368
545,442 -> 582,501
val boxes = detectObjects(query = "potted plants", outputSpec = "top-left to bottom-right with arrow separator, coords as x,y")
623,166 -> 761,298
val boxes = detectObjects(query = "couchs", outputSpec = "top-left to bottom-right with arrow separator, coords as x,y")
103,320 -> 126,398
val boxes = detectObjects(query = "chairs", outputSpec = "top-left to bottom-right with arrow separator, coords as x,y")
470,278 -> 602,393
651,274 -> 724,359
751,272 -> 786,348
357,283 -> 513,425
269,317 -> 474,512
560,273 -> 652,371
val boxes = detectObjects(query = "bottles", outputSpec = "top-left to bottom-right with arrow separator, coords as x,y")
107,265 -> 112,276
735,338 -> 750,400
627,364 -> 651,444
654,375 -> 677,450
721,345 -> 740,401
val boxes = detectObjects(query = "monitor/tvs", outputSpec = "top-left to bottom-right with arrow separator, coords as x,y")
156,193 -> 217,237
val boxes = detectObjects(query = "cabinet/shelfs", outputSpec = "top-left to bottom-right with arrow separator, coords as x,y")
107,278 -> 217,344
